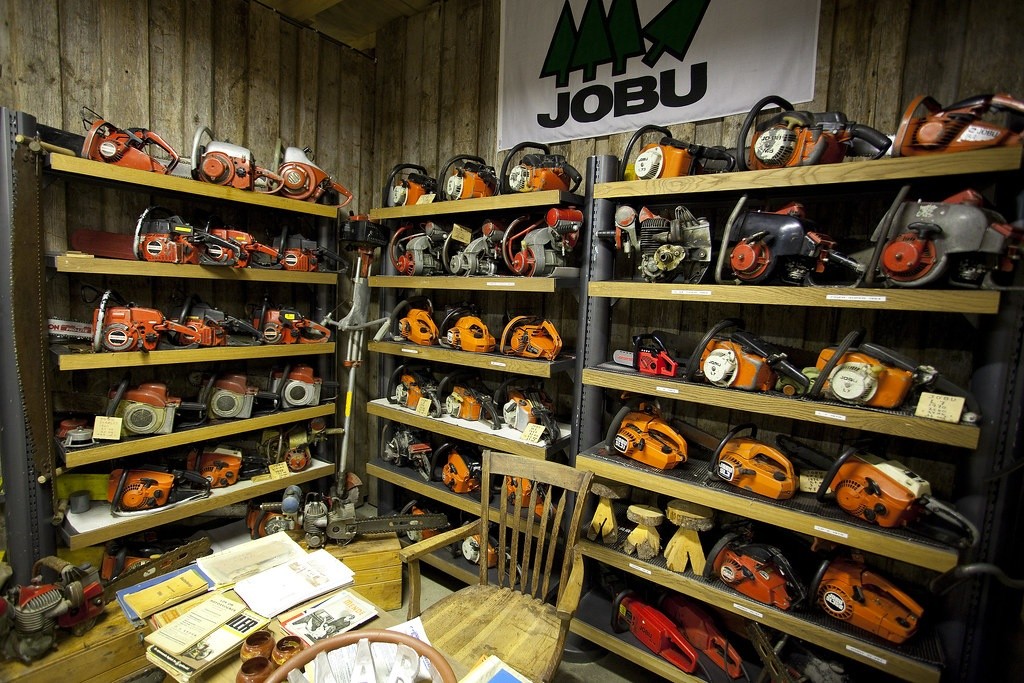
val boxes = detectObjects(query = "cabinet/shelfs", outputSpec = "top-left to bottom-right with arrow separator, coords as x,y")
0,105 -> 357,604
365,191 -> 586,602
569,146 -> 1024,683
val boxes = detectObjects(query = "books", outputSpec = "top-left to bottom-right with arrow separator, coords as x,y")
114,529 -> 377,683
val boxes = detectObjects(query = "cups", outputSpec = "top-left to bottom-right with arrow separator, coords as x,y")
69,490 -> 90,513
271,635 -> 305,681
239,630 -> 276,663
236,657 -> 273,683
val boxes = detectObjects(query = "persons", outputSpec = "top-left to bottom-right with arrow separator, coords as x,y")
323,614 -> 355,637
308,617 -> 335,641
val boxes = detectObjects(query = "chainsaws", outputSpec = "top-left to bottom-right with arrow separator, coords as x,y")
137,443 -> 268,489
52,371 -> 208,435
1,536 -> 212,664
70,204 -> 242,267
37,105 -> 182,175
231,418 -> 346,473
383,94 -> 1024,683
256,363 -> 339,410
152,125 -> 284,195
170,373 -> 283,422
157,291 -> 265,346
100,546 -> 171,580
232,297 -> 331,343
254,136 -> 355,209
245,485 -> 448,550
47,284 -> 201,354
192,223 -> 285,268
250,242 -> 350,275
53,464 -> 212,513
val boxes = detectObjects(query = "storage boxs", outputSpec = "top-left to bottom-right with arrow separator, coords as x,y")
284,520 -> 403,611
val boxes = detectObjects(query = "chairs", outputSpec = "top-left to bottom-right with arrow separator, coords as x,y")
398,450 -> 596,683
